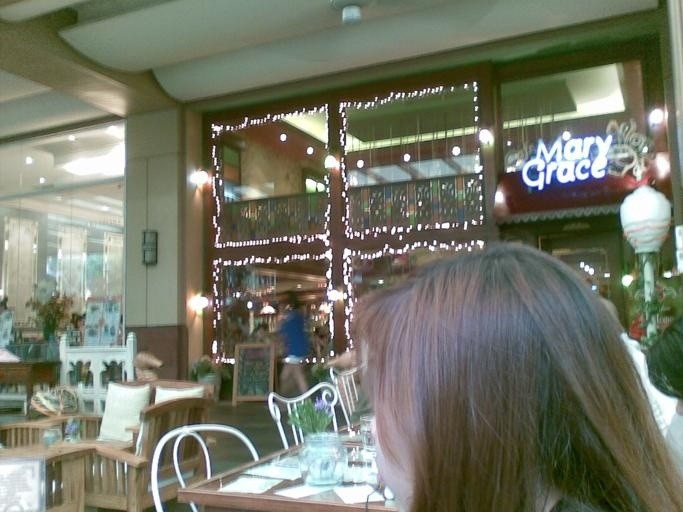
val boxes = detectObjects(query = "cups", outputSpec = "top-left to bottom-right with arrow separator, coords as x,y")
360,414 -> 379,452
297,432 -> 348,487
42,429 -> 63,448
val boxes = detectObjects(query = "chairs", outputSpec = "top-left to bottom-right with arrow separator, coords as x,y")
79,398 -> 208,512
152,424 -> 259,511
268,382 -> 339,450
330,364 -> 366,427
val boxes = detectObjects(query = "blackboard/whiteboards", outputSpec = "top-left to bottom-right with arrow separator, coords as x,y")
232,343 -> 274,401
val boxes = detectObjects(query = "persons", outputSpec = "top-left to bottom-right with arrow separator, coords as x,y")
352,242 -> 682,512
262,288 -> 309,400
644,314 -> 682,419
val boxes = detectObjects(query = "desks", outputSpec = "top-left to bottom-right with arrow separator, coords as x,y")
177,413 -> 408,512
1,443 -> 93,512
1,361 -> 60,419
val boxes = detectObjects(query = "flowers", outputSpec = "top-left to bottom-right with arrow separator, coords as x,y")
25,289 -> 75,329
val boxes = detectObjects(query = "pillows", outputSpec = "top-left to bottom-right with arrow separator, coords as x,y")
155,380 -> 205,407
97,383 -> 152,443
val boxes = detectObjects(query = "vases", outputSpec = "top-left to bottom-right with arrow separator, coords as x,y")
42,321 -> 55,341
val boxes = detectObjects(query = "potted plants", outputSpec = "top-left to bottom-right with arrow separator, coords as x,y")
194,361 -> 230,406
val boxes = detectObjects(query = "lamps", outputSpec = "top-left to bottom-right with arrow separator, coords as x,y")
140,229 -> 157,265
330,0 -> 366,25
